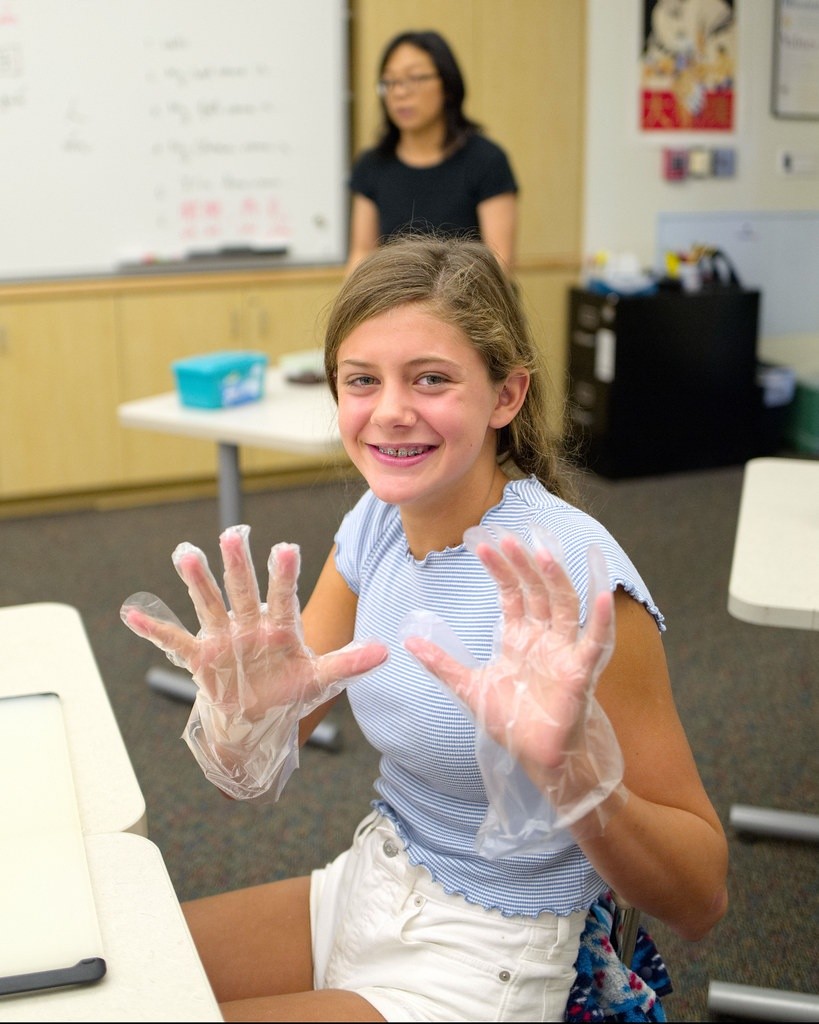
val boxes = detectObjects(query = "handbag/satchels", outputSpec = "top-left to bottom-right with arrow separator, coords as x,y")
679,249 -> 776,475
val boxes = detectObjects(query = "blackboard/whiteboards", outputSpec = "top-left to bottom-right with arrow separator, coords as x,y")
0,2 -> 361,287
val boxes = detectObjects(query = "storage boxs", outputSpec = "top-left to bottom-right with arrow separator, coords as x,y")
172,353 -> 268,408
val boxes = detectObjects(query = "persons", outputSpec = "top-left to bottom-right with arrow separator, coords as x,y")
343,30 -> 520,283
125,239 -> 730,1024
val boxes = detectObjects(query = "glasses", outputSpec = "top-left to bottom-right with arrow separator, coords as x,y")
374,69 -> 439,97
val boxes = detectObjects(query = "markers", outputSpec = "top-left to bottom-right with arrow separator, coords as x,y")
139,247 -> 293,267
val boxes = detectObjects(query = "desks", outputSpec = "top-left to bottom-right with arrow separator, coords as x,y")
1,602 -> 225,1023
115,351 -> 346,755
704,457 -> 819,1024
562,285 -> 764,483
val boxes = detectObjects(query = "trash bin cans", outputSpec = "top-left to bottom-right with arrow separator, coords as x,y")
737,376 -> 796,465
